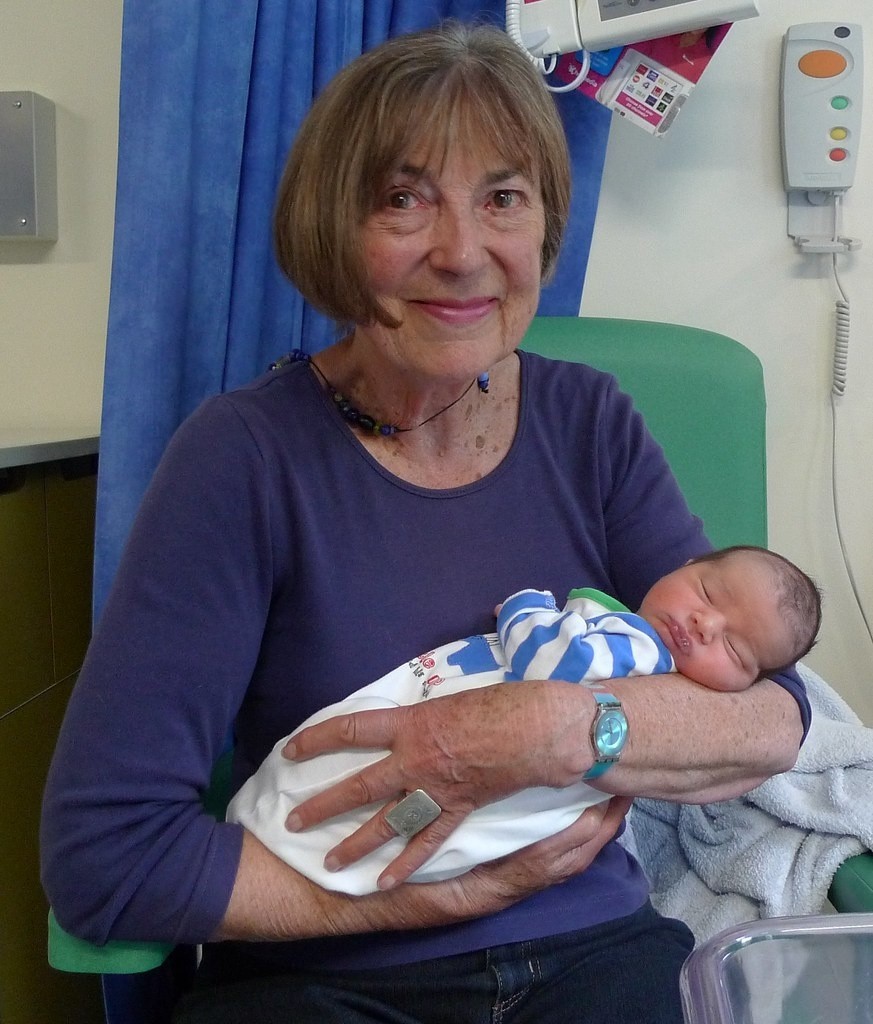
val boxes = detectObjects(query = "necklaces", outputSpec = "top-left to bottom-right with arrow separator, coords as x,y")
265,348 -> 491,439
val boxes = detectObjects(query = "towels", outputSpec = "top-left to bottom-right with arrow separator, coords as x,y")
616,662 -> 873,1024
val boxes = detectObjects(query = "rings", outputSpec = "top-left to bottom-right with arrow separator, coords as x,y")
383,783 -> 444,841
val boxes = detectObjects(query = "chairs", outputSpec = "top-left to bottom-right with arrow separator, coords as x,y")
47,310 -> 873,1024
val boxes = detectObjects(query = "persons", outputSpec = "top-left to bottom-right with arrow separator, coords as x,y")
225,543 -> 824,898
42,21 -> 813,1024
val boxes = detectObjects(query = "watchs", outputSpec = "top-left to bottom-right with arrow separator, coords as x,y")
579,675 -> 632,786
